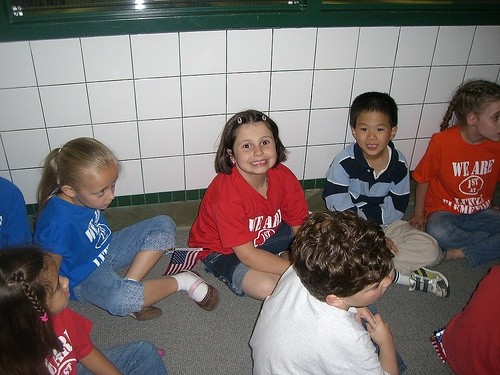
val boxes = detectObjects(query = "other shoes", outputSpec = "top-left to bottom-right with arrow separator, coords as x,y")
179,270 -> 220,311
128,305 -> 162,321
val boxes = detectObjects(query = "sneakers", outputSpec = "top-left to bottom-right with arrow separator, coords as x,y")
409,267 -> 450,298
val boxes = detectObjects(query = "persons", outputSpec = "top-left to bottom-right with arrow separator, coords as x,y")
321,91 -> 451,299
32,137 -> 221,321
248,209 -> 408,375
0,176 -> 169,375
407,79 -> 500,375
187,109 -> 310,301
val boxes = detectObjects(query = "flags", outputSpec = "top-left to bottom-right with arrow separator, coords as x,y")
428,330 -> 448,363
162,245 -> 203,276
